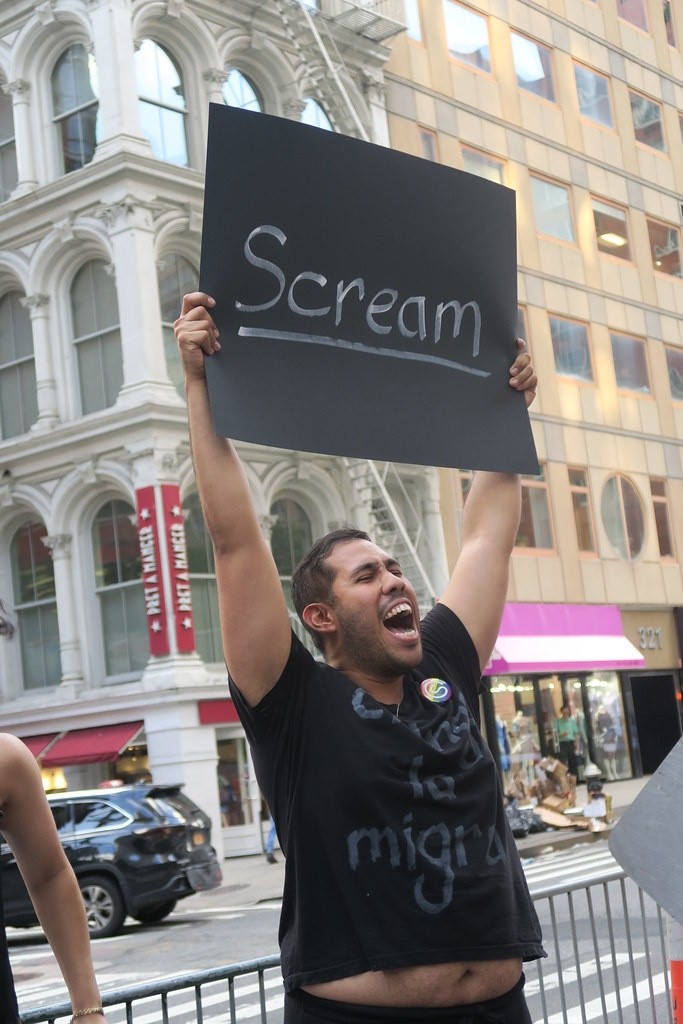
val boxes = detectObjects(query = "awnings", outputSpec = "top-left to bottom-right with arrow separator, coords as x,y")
19,720 -> 145,768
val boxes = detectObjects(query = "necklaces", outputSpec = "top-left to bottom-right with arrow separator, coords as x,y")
397,687 -> 404,719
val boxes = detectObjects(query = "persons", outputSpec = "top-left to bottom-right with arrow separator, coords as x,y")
554,706 -> 580,785
264,810 -> 277,864
172,290 -> 548,1024
0,606 -> 111,1024
594,705 -> 620,781
512,710 -> 541,787
494,708 -> 512,805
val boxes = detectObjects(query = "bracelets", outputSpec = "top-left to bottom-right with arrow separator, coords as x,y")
70,1007 -> 104,1023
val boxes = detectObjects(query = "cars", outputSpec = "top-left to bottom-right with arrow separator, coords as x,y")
1,783 -> 223,940
